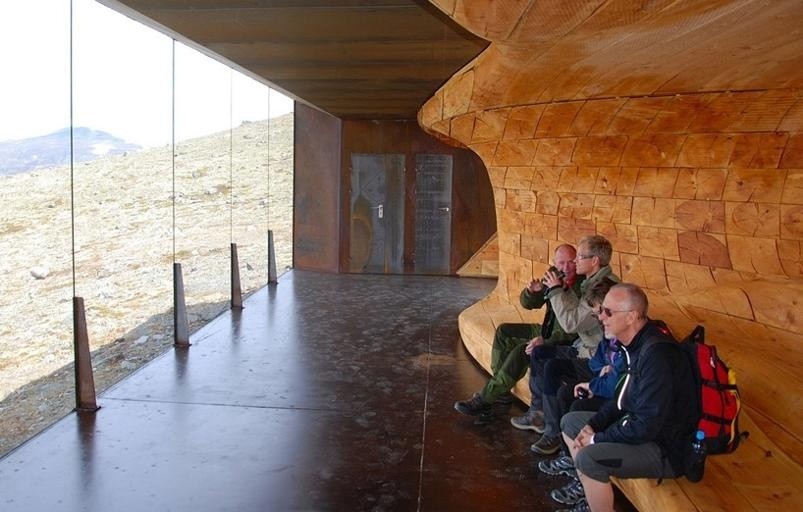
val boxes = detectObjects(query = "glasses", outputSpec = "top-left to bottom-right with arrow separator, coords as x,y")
599,306 -> 634,317
576,255 -> 594,260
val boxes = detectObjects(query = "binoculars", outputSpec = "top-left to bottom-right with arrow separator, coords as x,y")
541,266 -> 565,290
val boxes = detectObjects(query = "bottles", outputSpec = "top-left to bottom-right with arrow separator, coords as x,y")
693,432 -> 706,458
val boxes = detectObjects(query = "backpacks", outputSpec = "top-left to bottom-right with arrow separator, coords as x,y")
635,320 -> 741,454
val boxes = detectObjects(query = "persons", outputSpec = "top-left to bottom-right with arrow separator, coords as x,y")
453,244 -> 587,417
539,277 -> 698,512
512,236 -> 622,454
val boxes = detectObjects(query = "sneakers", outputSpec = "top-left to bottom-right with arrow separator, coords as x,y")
454,391 -> 512,416
510,411 -> 591,512
